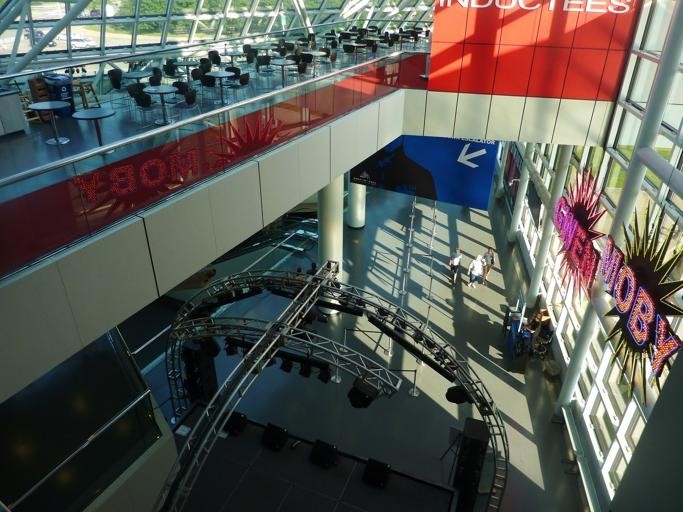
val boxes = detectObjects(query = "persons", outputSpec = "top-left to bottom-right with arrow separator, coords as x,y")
180,333 -> 220,406
448,247 -> 463,290
483,247 -> 493,283
523,313 -> 541,354
467,255 -> 486,288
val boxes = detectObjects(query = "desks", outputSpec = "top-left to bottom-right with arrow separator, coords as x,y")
0,87 -> 25,139
501,306 -> 522,337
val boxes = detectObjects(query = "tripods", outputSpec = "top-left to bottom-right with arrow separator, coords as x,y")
438,440 -> 460,485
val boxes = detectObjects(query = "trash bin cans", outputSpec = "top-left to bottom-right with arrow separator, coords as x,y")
42,73 -> 76,118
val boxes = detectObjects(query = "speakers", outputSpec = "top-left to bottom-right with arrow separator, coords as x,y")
218,411 -> 248,436
262,421 -> 289,454
362,457 -> 398,488
304,438 -> 337,474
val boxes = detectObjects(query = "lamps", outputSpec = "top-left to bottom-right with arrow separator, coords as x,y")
180,276 -> 469,410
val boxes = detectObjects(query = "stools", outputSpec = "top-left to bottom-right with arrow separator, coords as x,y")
532,335 -> 551,360
